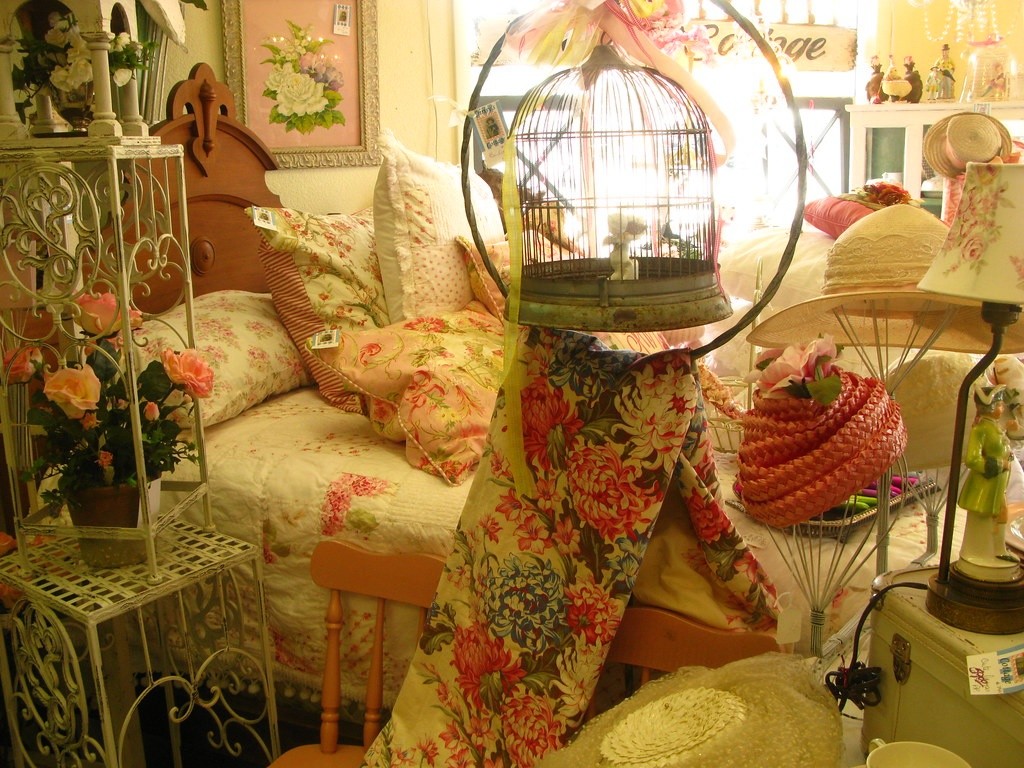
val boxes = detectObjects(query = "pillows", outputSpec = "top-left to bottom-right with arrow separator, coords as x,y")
719,230 -> 836,311
593,331 -> 669,355
245,205 -> 390,416
305,301 -> 504,487
374,129 -> 505,325
114,290 -> 315,428
803,196 -> 876,239
456,235 -> 560,325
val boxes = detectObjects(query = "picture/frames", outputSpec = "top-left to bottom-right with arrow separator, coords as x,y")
221,0 -> 382,170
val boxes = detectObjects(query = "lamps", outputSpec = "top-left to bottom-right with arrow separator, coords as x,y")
917,161 -> 1024,633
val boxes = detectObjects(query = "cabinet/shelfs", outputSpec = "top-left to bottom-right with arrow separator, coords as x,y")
845,102 -> 1024,207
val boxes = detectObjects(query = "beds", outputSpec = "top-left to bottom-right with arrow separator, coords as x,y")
80,61 -> 1023,722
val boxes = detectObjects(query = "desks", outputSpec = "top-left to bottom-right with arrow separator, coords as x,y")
1,515 -> 280,768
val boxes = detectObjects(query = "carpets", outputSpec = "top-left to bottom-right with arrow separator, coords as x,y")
4,291 -> 214,520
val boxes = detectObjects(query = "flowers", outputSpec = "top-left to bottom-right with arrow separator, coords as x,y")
12,12 -> 160,110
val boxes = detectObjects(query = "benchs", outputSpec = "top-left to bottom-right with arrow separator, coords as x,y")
267,541 -> 784,768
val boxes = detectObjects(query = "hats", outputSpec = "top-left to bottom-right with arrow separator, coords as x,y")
924,112 -> 1012,183
733,334 -> 908,528
746,204 -> 1024,354
879,348 -> 990,475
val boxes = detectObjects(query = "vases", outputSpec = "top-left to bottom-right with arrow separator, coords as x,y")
58,471 -> 162,568
49,79 -> 94,138
